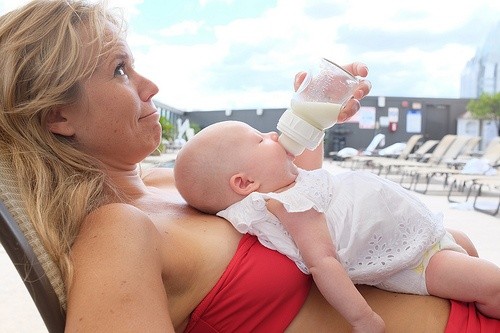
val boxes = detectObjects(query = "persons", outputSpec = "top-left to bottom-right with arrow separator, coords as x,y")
173,120 -> 500,333
0,1 -> 500,332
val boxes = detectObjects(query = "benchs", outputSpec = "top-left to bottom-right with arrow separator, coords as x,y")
328,132 -> 499,220
0,137 -> 83,333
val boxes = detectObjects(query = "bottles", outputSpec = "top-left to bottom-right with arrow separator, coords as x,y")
275,58 -> 361,157
